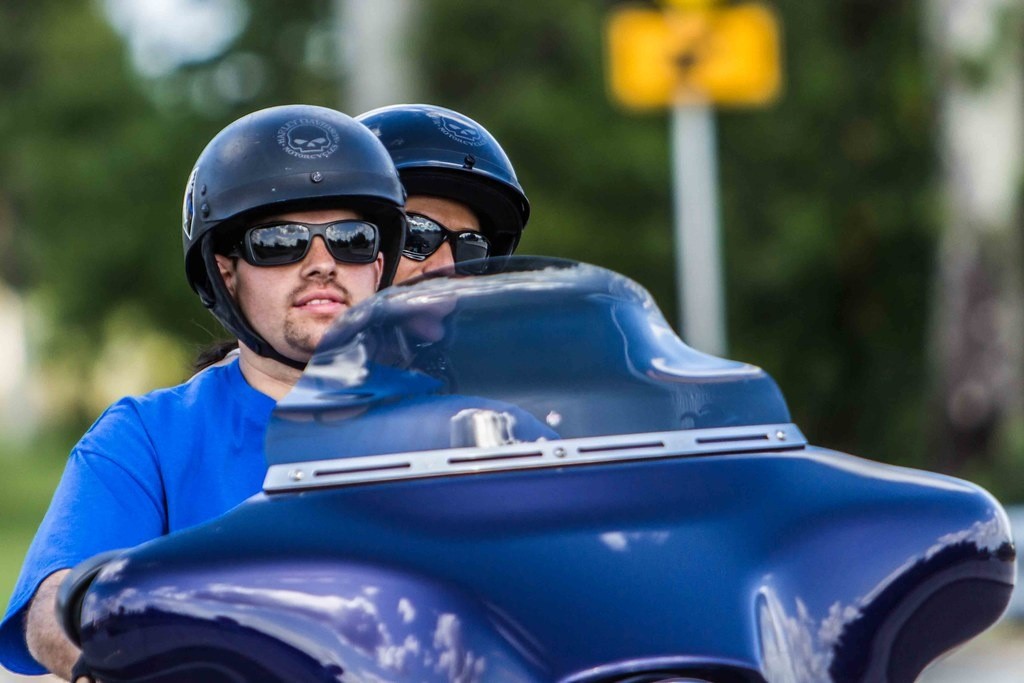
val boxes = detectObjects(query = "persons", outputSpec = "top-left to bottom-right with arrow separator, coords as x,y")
347,105 -> 535,394
0,102 -> 565,683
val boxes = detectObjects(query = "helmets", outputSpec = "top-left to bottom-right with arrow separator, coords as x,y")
354,105 -> 531,275
181,104 -> 407,317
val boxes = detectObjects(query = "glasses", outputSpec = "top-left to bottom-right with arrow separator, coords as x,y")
400,208 -> 492,277
213,220 -> 381,268
